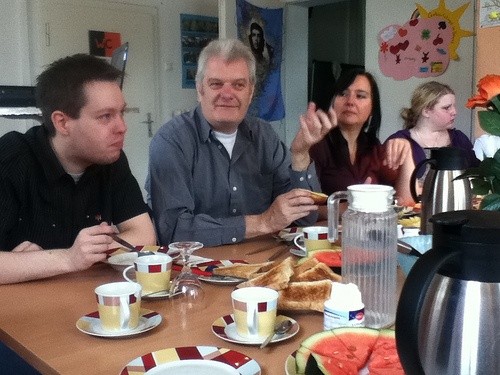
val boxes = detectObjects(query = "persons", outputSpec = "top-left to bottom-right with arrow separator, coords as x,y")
144,39 -> 337,248
0,53 -> 156,375
310,68 -> 416,203
385,82 -> 479,169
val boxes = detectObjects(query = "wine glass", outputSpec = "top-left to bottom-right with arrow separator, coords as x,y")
168,241 -> 207,314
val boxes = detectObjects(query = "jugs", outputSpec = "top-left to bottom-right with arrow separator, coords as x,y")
410,147 -> 473,235
327,183 -> 398,329
395,209 -> 500,375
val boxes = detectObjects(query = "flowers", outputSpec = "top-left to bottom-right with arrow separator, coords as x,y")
451,73 -> 500,212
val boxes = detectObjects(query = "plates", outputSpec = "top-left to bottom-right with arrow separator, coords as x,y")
273,226 -> 303,242
75,307 -> 163,339
284,347 -> 298,374
290,244 -> 305,258
186,259 -> 253,286
108,251 -> 167,272
394,206 -> 421,219
120,345 -> 262,375
141,280 -> 181,300
398,232 -> 433,256
211,313 -> 299,345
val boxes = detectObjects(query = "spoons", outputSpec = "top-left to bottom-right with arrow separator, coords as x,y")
86,214 -> 155,257
260,319 -> 292,351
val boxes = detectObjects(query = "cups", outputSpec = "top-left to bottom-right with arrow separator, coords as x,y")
230,287 -> 279,340
122,255 -> 173,294
322,300 -> 366,330
293,226 -> 328,254
94,282 -> 143,331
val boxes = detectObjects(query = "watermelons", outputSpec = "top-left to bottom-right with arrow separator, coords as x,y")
293,327 -> 407,375
308,248 -> 389,267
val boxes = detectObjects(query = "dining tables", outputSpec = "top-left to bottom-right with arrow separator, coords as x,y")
0,203 -> 405,375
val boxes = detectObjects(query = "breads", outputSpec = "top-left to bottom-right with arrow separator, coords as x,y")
212,256 -> 342,312
309,191 -> 328,201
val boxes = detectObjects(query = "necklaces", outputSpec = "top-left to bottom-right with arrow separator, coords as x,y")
416,131 -> 438,147
